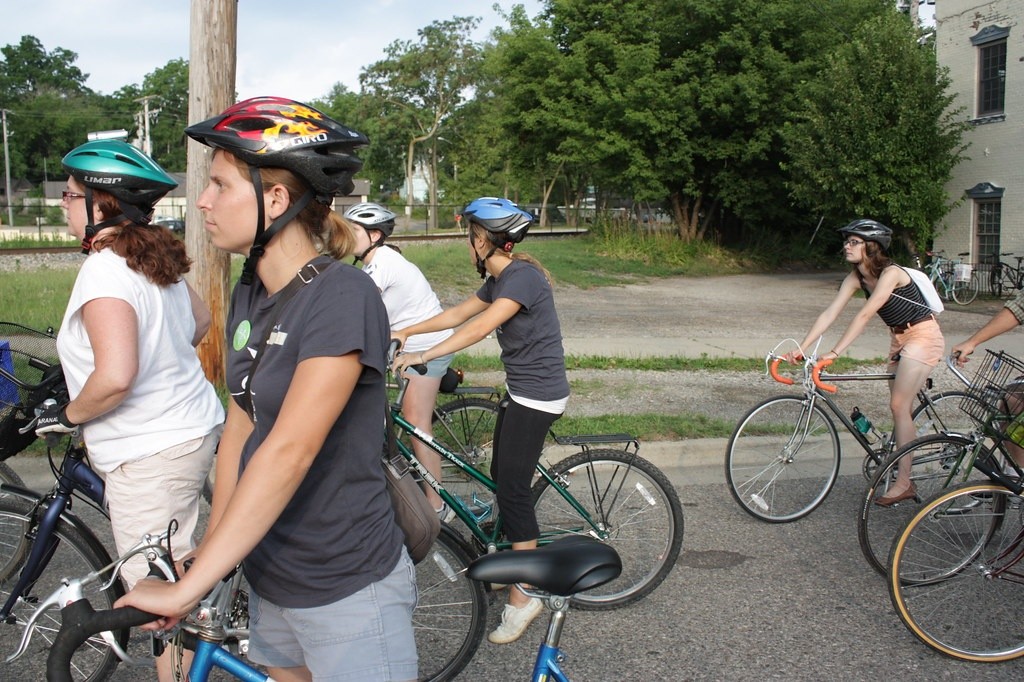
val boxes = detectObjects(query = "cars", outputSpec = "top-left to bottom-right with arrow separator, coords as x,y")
154,221 -> 184,232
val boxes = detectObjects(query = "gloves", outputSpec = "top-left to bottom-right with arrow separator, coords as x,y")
35,402 -> 80,439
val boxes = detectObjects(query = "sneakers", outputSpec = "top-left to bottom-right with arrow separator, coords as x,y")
490,582 -> 508,590
488,593 -> 543,644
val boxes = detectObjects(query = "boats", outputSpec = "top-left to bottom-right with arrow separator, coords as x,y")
557,197 -> 626,221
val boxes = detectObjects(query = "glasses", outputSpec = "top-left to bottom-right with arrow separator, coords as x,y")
62,189 -> 92,202
843,238 -> 865,248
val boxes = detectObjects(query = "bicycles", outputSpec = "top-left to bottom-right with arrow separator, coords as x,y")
0,318 -> 486,682
917,249 -> 980,305
986,252 -> 1024,297
383,338 -> 684,612
8,485 -> 622,682
723,335 -> 1011,524
856,344 -> 1024,662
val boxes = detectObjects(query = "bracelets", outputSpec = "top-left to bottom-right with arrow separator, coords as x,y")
420,351 -> 426,364
831,350 -> 838,356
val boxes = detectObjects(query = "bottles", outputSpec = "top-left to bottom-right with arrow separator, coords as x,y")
850,406 -> 882,444
1006,412 -> 1024,449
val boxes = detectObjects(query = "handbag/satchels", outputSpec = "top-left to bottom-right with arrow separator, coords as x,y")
381,440 -> 440,566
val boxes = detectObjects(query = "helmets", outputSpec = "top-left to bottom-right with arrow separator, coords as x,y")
343,202 -> 398,236
458,195 -> 535,242
837,219 -> 892,250
61,136 -> 178,208
184,95 -> 370,196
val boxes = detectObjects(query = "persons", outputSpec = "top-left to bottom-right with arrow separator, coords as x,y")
785,219 -> 944,505
390,197 -> 569,643
114,95 -> 421,682
951,285 -> 1024,506
32,138 -> 222,682
341,202 -> 454,529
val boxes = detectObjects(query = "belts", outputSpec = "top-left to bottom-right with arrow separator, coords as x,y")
890,315 -> 932,334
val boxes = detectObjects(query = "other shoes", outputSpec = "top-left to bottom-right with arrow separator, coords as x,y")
436,502 -> 457,525
970,490 -> 1024,505
875,481 -> 917,506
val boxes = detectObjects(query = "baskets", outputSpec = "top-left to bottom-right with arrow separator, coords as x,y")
958,348 -> 1024,448
0,322 -> 62,422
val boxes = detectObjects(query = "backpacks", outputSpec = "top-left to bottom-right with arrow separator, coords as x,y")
878,262 -> 944,315
